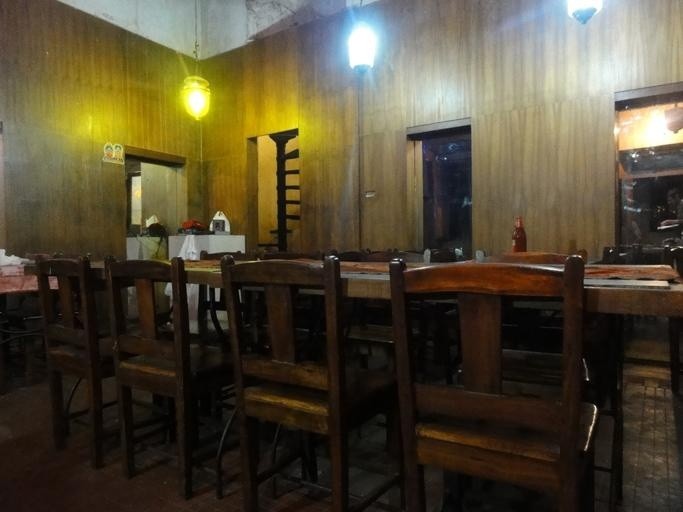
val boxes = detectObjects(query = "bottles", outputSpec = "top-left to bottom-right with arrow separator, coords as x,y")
512,216 -> 527,253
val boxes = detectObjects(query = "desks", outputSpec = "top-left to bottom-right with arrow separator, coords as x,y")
183,258 -> 682,452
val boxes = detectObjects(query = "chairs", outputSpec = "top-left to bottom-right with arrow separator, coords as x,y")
33,250 -> 136,470
386,250 -> 593,512
98,253 -> 230,497
214,253 -> 402,510
